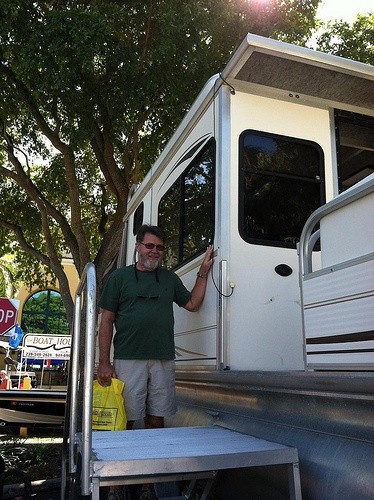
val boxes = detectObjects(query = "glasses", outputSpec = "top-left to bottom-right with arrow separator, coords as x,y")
138,241 -> 166,251
0,374 -> 3,376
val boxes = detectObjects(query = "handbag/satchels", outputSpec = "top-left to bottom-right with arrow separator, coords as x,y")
92,378 -> 127,431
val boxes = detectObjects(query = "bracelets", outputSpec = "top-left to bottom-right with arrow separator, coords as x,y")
197,271 -> 207,278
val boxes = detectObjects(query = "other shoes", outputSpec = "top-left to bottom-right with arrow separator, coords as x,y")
140,487 -> 153,500
107,489 -> 122,500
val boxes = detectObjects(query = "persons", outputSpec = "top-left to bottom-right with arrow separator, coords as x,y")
96,224 -> 214,500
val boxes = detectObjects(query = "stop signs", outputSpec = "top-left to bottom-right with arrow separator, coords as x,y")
0,298 -> 17,336
